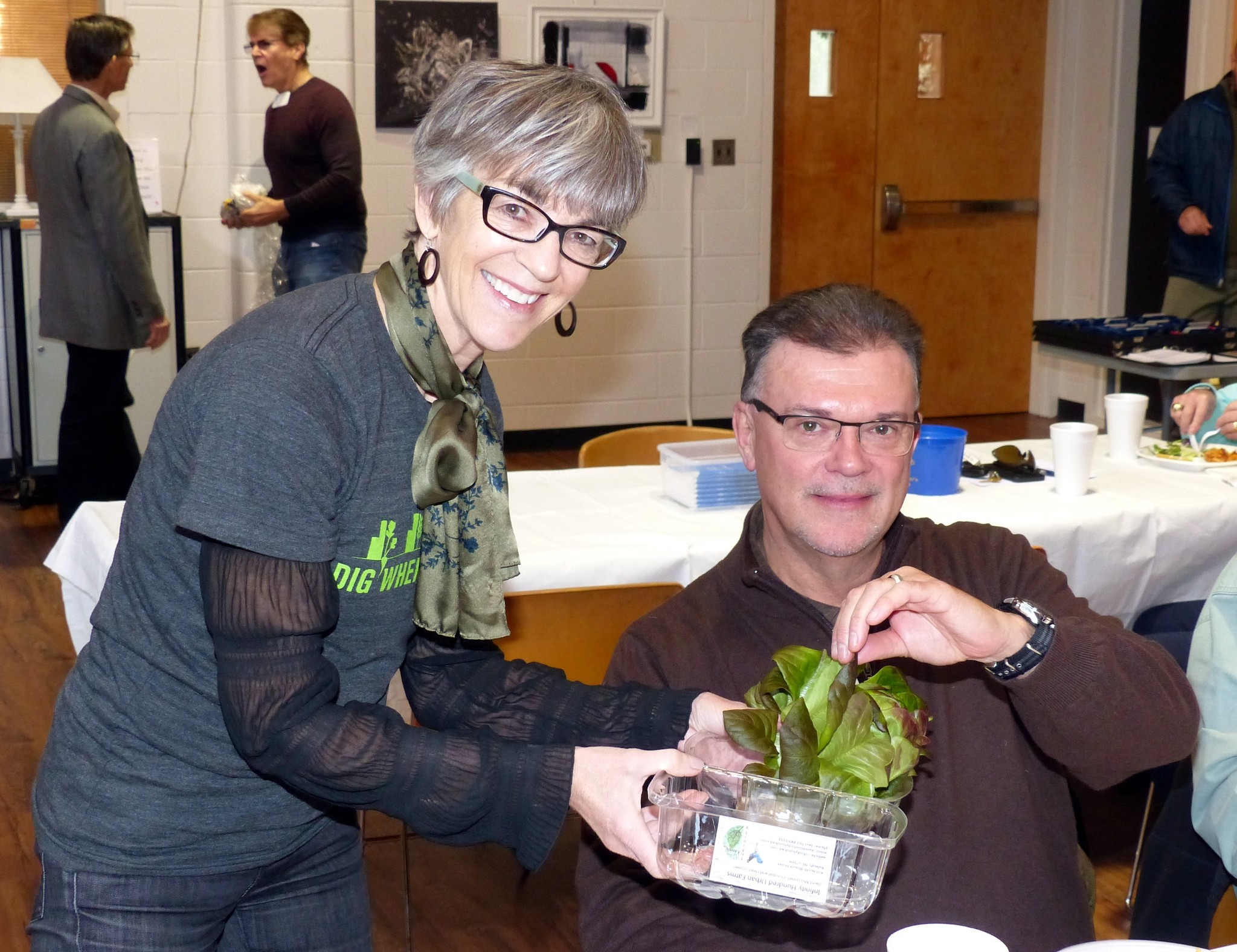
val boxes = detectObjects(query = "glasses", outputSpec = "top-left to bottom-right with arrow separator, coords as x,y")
114,44 -> 140,63
243,37 -> 292,52
745,392 -> 923,458
446,158 -> 628,272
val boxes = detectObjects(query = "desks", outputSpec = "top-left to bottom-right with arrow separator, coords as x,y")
1033,316 -> 1236,444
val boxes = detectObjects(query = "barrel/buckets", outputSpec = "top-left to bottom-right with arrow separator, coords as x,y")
900,421 -> 968,496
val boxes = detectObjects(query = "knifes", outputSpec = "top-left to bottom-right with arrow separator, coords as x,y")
1189,430 -> 1200,456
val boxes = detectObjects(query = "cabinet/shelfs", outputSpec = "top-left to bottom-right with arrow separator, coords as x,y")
0,202 -> 188,508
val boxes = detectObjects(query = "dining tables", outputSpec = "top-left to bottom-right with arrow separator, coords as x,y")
43,428 -> 1235,951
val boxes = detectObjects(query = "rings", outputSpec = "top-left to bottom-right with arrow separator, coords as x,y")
1233,422 -> 1237,430
887,573 -> 902,584
1171,403 -> 1183,411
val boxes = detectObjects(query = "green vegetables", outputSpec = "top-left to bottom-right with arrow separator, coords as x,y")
722,644 -> 935,817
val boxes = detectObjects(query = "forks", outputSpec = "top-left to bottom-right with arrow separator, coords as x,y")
1200,425 -> 1225,451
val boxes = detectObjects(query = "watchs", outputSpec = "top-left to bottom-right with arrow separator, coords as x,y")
982,596 -> 1056,681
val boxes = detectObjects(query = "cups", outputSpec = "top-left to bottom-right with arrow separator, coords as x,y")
1049,419 -> 1100,498
1105,391 -> 1149,462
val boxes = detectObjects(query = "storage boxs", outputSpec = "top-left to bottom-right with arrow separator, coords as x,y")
657,437 -> 760,507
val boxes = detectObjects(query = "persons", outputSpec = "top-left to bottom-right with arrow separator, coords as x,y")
218,5 -> 371,301
574,283 -> 1203,950
1144,43 -> 1237,440
1169,377 -> 1237,447
29,12 -> 172,532
1182,536 -> 1235,952
21,54 -> 784,952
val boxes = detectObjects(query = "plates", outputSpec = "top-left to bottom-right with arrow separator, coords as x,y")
1138,439 -> 1237,472
1118,345 -> 1213,366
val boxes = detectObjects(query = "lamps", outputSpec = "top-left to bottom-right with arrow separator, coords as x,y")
0,57 -> 64,217
